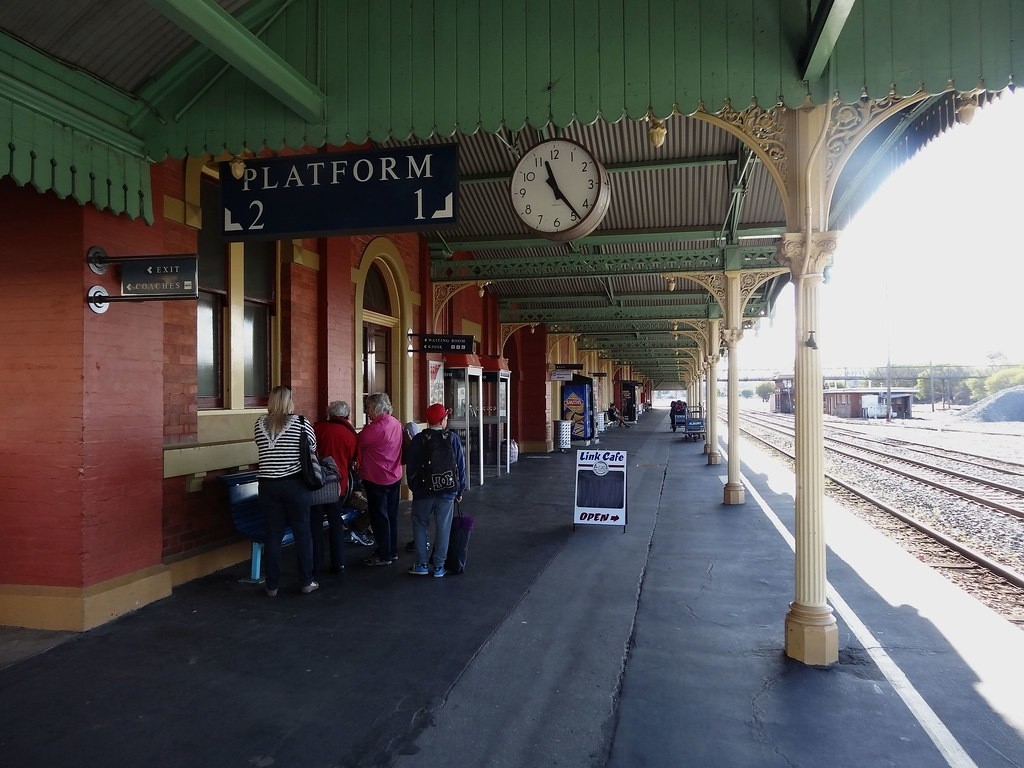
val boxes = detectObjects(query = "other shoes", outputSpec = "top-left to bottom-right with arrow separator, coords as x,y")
265,585 -> 279,598
334,560 -> 346,570
301,580 -> 320,594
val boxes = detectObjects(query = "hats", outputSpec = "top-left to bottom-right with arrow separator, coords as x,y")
427,403 -> 451,424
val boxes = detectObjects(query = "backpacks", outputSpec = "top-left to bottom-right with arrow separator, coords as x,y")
415,428 -> 462,497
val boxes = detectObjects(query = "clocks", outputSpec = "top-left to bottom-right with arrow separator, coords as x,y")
506,137 -> 612,244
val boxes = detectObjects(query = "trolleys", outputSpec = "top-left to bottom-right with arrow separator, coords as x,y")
669,402 -> 688,432
682,405 -> 706,442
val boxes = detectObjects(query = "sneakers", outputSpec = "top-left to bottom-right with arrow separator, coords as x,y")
432,566 -> 448,578
371,550 -> 400,560
347,528 -> 375,546
407,561 -> 429,576
361,557 -> 393,568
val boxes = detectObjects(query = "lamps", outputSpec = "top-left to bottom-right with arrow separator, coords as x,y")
530,325 -> 535,334
649,118 -> 667,147
667,278 -> 677,292
229,153 -> 248,180
673,323 -> 681,382
477,286 -> 485,297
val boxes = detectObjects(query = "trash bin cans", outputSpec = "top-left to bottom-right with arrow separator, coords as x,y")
598,412 -> 605,431
552,420 -> 573,452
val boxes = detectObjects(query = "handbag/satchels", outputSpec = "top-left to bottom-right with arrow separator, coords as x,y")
299,415 -> 324,492
389,414 -> 414,466
428,496 -> 476,576
302,454 -> 341,506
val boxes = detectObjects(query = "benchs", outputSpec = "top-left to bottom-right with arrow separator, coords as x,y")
217,467 -> 363,584
598,419 -> 617,433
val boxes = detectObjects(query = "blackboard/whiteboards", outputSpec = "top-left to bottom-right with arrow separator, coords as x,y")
574,449 -> 628,526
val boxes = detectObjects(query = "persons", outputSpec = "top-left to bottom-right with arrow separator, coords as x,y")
254,388 -> 319,596
406,403 -> 466,577
345,489 -> 374,548
357,392 -> 404,566
313,401 -> 356,579
670,401 -> 688,431
608,403 -> 632,428
